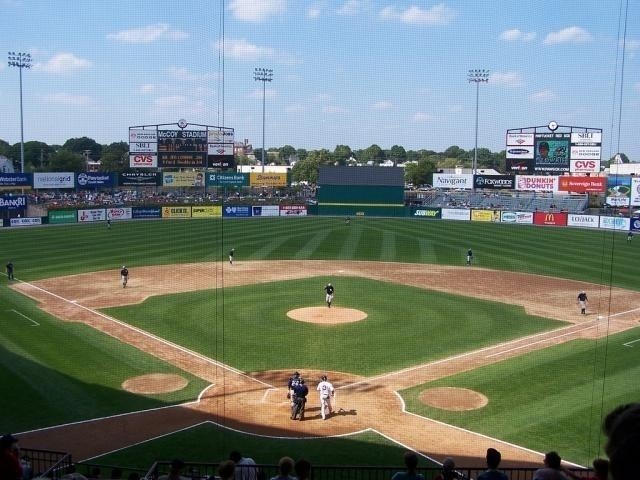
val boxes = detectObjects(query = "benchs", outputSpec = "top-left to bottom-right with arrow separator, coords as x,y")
421,186 -> 594,218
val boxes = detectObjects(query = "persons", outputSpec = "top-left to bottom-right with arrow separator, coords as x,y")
316,375 -> 335,420
120,265 -> 129,288
627,232 -> 634,246
345,216 -> 351,226
287,371 -> 300,407
407,183 -> 570,213
577,291 -> 588,314
0,404 -> 639,480
289,377 -> 308,420
324,282 -> 334,307
108,218 -> 112,227
29,182 -> 319,208
6,261 -> 14,280
228,248 -> 234,264
536,141 -> 552,163
465,247 -> 472,265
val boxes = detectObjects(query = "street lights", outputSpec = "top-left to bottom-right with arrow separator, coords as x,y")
466,67 -> 492,176
6,50 -> 35,173
253,67 -> 275,171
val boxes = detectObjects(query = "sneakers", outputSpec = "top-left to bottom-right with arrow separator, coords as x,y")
291,411 -> 332,420
